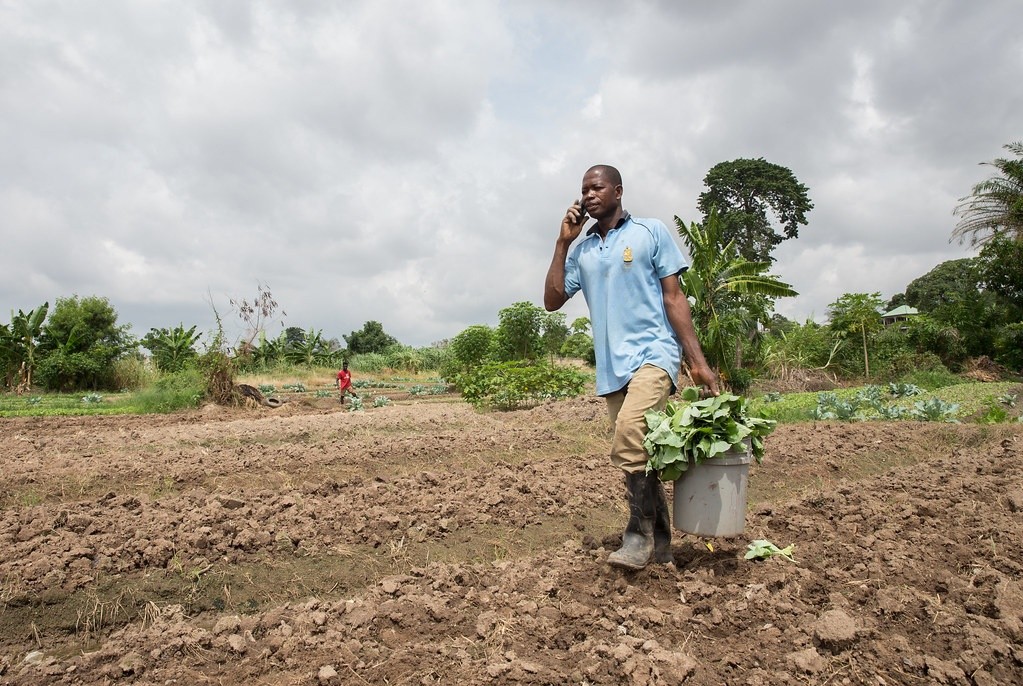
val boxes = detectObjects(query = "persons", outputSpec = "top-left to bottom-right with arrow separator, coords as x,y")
336,362 -> 358,404
543,165 -> 720,570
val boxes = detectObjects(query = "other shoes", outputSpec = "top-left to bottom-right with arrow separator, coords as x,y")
356,396 -> 359,399
340,400 -> 344,404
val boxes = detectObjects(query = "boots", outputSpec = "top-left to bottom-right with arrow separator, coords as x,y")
654,483 -> 675,565
607,470 -> 656,570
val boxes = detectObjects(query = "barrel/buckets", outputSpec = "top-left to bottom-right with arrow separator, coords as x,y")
672,435 -> 753,540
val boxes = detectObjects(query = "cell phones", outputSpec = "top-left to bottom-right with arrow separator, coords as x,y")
575,201 -> 587,225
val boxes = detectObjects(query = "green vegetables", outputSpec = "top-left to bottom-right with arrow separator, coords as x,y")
643,385 -> 777,481
743,539 -> 798,563
